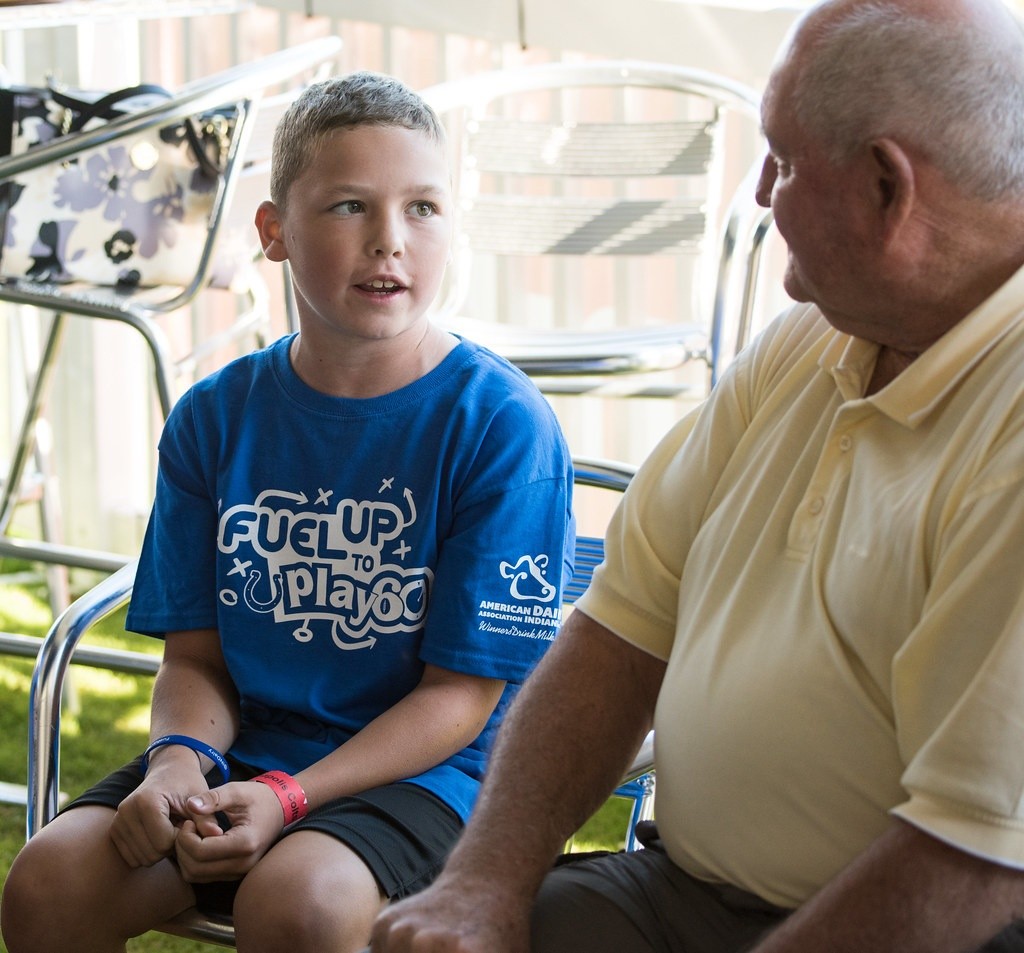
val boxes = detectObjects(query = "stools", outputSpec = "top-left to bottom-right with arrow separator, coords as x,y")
1,33 -> 345,811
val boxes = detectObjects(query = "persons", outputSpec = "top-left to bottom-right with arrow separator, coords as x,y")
366,2 -> 1024,951
1,75 -> 574,951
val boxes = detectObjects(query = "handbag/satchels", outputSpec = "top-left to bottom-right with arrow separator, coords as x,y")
0,86 -> 244,289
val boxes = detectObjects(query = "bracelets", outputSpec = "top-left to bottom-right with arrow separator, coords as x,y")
140,735 -> 231,786
249,770 -> 308,833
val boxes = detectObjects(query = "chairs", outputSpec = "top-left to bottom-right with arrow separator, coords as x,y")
279,64 -> 779,402
28,462 -> 662,943
